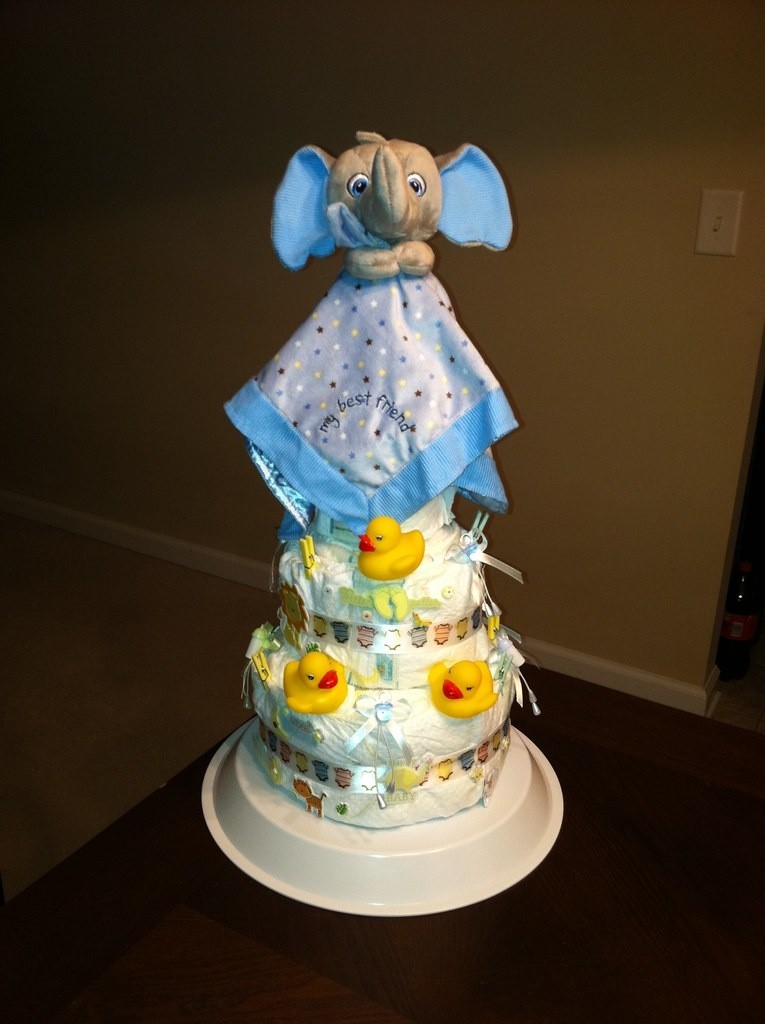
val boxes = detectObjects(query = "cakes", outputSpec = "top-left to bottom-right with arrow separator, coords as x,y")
237,481 -> 544,831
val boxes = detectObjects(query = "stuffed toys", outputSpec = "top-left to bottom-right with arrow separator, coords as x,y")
222,131 -> 519,541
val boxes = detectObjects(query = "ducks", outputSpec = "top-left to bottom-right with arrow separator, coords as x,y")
359,516 -> 425,582
429,660 -> 497,718
283,652 -> 347,716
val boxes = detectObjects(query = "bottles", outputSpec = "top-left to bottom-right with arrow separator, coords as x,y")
715,560 -> 759,680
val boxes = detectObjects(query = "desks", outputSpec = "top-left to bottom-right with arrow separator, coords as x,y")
0,663 -> 765,1024
